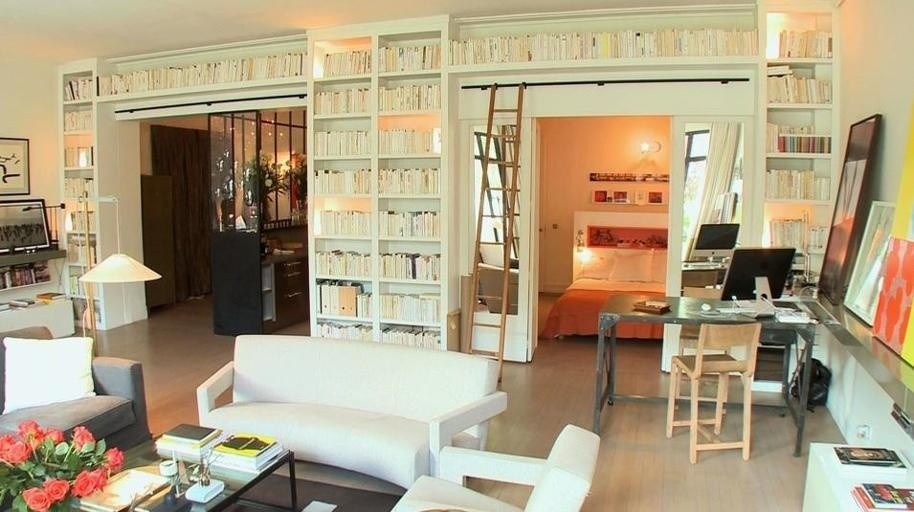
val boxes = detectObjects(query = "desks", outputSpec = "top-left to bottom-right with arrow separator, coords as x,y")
588,292 -> 823,458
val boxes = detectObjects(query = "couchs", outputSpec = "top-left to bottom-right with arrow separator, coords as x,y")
195,333 -> 509,492
0,324 -> 153,453
390,423 -> 603,512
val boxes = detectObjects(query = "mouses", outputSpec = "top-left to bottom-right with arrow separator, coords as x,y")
702,303 -> 712,312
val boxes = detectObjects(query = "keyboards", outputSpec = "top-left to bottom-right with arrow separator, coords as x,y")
715,306 -> 795,314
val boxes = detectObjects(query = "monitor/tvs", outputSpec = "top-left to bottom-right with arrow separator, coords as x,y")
690,224 -> 740,266
720,248 -> 797,318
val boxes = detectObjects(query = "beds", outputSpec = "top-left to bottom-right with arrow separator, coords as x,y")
558,195 -> 669,341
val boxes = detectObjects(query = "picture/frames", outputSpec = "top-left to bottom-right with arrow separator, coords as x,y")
0,199 -> 51,257
611,188 -> 631,204
591,188 -> 609,205
647,191 -> 664,205
0,138 -> 31,198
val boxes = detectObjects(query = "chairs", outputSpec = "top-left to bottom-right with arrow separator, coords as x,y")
665,321 -> 764,465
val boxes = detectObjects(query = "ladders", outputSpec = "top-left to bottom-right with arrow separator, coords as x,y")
502,126 -> 522,259
464,83 -> 525,381
474,131 -> 517,261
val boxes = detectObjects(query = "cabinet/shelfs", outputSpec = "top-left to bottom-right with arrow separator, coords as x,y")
760,4 -> 843,396
0,249 -> 75,339
309,12 -> 459,361
449,0 -> 760,386
53,32 -> 309,333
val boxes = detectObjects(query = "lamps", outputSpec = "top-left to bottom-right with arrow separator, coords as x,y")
75,190 -> 162,357
627,127 -> 662,155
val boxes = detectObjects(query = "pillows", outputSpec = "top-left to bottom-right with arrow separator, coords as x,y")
2,335 -> 99,412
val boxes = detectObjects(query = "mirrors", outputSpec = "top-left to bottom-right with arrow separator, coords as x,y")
817,113 -> 882,306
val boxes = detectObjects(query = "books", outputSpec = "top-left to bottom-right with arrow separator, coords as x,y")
381,326 -> 441,350
0,293 -> 67,311
324,49 -> 371,77
66,178 -> 94,198
380,45 -> 442,72
378,83 -> 441,113
768,124 -> 831,153
766,168 -> 830,200
72,299 -> 101,322
0,263 -> 50,289
315,249 -> 372,277
833,444 -> 907,479
779,30 -> 832,58
314,168 -> 371,193
851,483 -> 914,512
379,209 -> 439,237
71,466 -> 176,512
64,79 -> 92,101
767,65 -> 832,104
380,293 -> 440,324
70,274 -> 98,296
314,129 -> 370,155
65,145 -> 93,167
207,433 -> 291,483
64,110 -> 92,130
71,210 -> 95,232
767,211 -> 827,251
68,239 -> 96,264
314,87 -> 370,114
317,322 -> 373,343
155,423 -> 231,465
317,279 -> 372,319
321,210 -> 371,235
378,129 -> 441,156
379,167 -> 440,196
96,51 -> 306,96
379,252 -> 440,280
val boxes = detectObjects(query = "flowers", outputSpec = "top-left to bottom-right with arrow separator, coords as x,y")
0,419 -> 125,512
